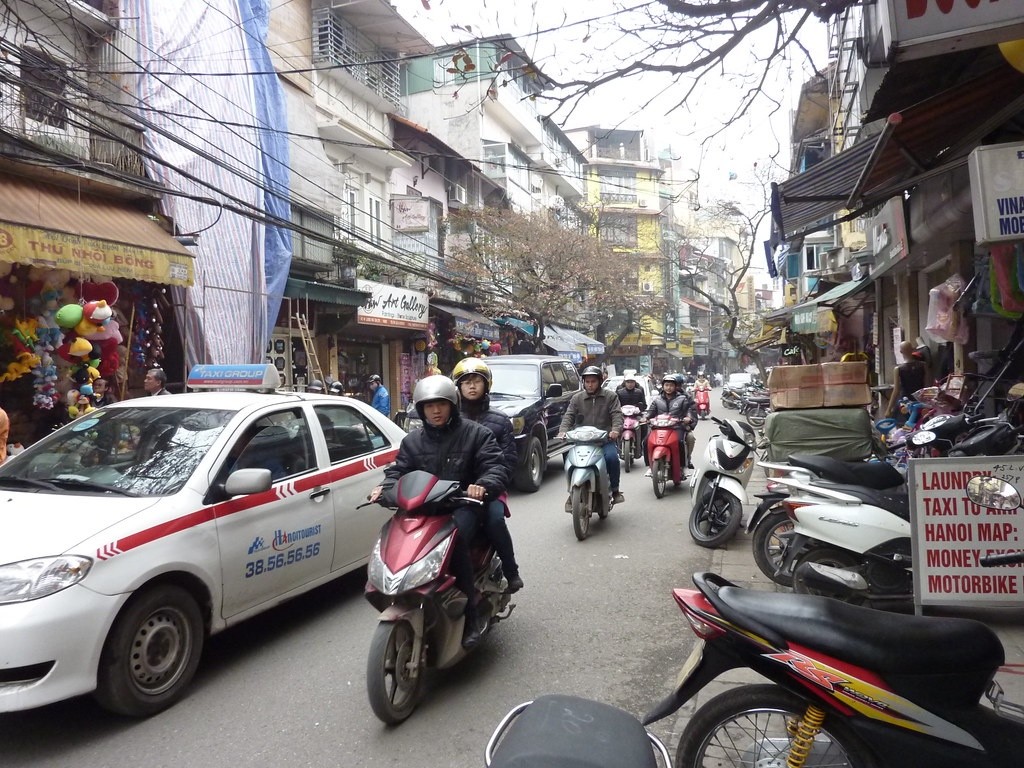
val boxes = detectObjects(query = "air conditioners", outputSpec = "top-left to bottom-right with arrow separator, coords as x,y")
642,282 -> 653,292
449,184 -> 466,204
34,134 -> 88,162
826,248 -> 851,269
555,157 -> 563,167
640,199 -> 647,206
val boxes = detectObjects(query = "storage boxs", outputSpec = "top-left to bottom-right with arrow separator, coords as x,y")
759,404 -> 877,462
768,362 -> 872,409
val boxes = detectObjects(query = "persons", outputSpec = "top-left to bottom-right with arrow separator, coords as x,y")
451,358 -> 523,592
369,376 -> 508,648
914,337 -> 932,368
307,380 -> 325,393
84,378 -> 122,459
327,381 -> 344,396
368,375 -> 391,417
145,369 -> 171,397
615,368 -> 712,480
558,366 -> 624,512
885,341 -> 928,418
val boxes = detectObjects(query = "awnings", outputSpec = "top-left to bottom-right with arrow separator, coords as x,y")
763,63 -> 1024,323
549,323 -> 606,355
0,172 -> 196,289
429,302 -> 500,340
284,278 -> 373,308
497,317 -> 583,364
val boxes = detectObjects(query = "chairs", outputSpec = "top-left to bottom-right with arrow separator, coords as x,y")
254,425 -> 290,450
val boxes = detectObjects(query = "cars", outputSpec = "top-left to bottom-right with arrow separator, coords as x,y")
401,354 -> 587,491
0,365 -> 417,722
723,373 -> 757,405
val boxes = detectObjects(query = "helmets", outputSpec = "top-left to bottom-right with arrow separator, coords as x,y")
697,371 -> 706,378
329,381 -> 344,392
366,374 -> 381,382
412,374 -> 458,422
662,374 -> 683,382
580,366 -> 603,378
452,357 -> 493,394
307,379 -> 323,391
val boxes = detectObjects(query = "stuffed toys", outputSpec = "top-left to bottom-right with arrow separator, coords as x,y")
0,260 -> 131,457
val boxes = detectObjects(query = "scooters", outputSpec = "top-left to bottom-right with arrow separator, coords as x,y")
688,415 -> 771,548
365,471 -> 514,730
613,401 -> 646,473
636,412 -> 688,501
554,425 -> 617,543
723,382 -> 771,426
690,385 -> 712,421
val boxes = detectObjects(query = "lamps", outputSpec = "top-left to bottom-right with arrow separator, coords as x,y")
413,174 -> 418,187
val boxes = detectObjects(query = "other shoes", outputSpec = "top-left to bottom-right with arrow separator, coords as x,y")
463,625 -> 482,647
612,491 -> 625,503
503,570 -> 523,593
645,468 -> 653,477
565,496 -> 573,513
687,461 -> 694,469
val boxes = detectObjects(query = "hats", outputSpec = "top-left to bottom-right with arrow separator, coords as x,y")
624,373 -> 636,381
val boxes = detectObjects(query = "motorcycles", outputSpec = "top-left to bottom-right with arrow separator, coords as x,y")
643,475 -> 1024,767
483,693 -> 674,767
765,381 -> 1024,621
744,412 -> 988,587
